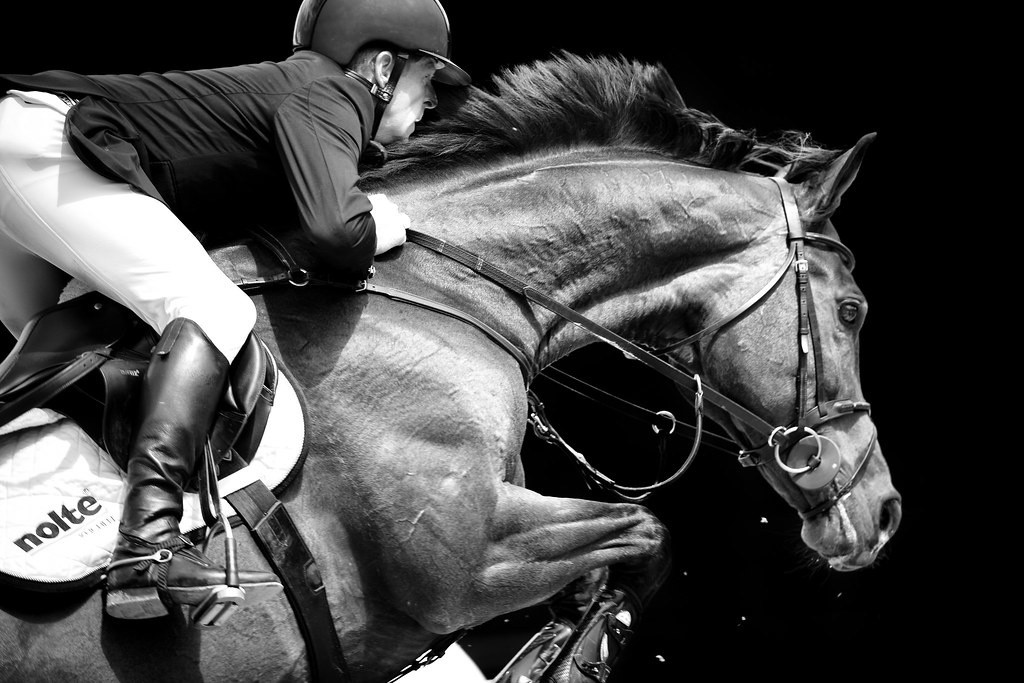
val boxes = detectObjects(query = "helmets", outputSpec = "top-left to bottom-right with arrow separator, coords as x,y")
292,0 -> 472,88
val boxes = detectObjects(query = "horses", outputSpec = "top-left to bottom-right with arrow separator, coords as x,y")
0,51 -> 903,683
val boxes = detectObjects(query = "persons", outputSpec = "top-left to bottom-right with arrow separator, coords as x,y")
0,0 -> 470,619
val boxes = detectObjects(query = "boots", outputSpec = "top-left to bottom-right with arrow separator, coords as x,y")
103,315 -> 285,620
